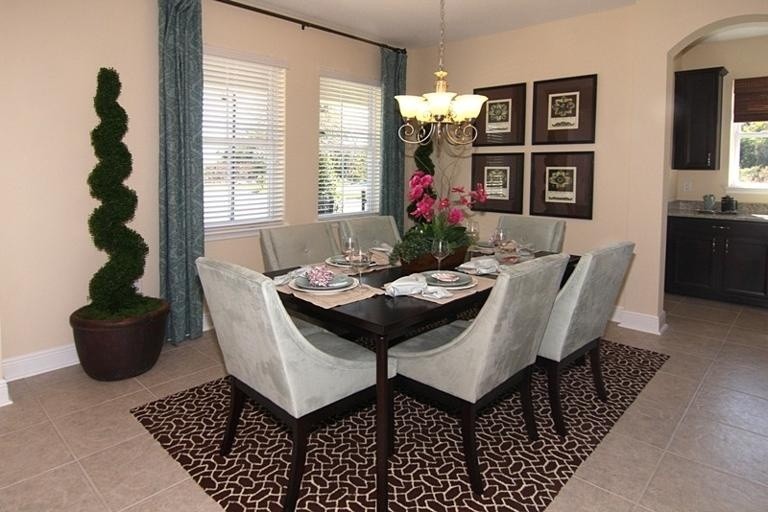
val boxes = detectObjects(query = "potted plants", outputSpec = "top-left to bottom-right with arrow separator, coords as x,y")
69,66 -> 170,382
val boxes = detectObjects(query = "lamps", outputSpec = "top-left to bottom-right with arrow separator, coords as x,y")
394,0 -> 489,147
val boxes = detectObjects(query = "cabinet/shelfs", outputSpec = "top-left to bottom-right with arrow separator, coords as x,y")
665,216 -> 768,309
672,66 -> 729,170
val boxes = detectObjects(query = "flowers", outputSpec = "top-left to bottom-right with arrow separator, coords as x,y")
388,171 -> 488,264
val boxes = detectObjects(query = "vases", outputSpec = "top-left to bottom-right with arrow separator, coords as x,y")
401,247 -> 468,275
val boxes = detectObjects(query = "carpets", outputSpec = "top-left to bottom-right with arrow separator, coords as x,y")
132,305 -> 671,512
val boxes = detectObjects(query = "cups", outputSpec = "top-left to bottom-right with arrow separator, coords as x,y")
721,196 -> 739,213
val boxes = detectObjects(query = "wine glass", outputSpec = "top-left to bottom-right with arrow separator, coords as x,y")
349,248 -> 371,291
495,227 -> 508,247
466,221 -> 480,251
342,235 -> 359,262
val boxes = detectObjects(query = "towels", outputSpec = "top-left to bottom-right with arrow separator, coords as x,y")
455,259 -> 498,275
384,273 -> 454,300
272,267 -> 312,286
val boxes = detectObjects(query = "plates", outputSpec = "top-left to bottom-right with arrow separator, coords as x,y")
325,247 -> 395,268
419,239 -> 536,290
287,277 -> 359,295
295,274 -> 354,290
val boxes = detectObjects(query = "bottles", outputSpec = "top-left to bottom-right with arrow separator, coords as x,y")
703,193 -> 716,210
514,229 -> 537,259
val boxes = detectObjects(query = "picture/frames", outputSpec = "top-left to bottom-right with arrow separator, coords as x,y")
471,74 -> 600,221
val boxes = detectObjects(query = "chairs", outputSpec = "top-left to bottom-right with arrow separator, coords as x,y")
195,256 -> 398,512
338,215 -> 402,253
257,215 -> 340,272
469,241 -> 636,436
388,253 -> 571,495
497,215 -> 567,253
